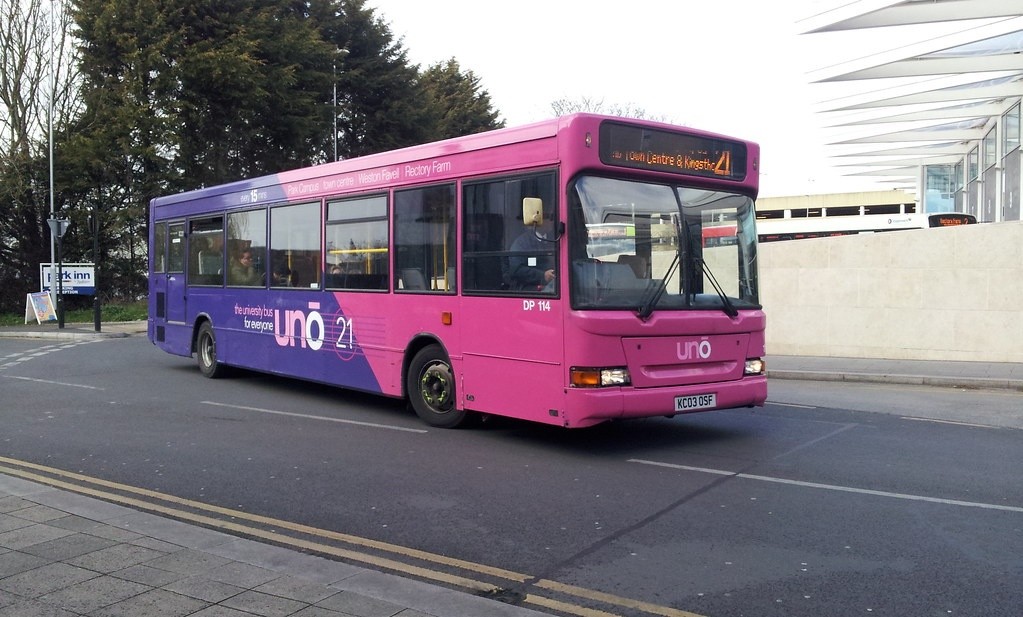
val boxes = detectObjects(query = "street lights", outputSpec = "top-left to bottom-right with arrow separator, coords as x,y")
333,48 -> 350,162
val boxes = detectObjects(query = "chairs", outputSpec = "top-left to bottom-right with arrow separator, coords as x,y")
198,250 -> 222,274
330,265 -> 345,275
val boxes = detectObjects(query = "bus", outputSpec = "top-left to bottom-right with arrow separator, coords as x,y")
701,211 -> 976,248
147,112 -> 767,428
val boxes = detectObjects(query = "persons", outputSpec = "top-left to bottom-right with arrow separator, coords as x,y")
509,214 -> 556,291
170,203 -> 507,291
630,253 -> 652,282
